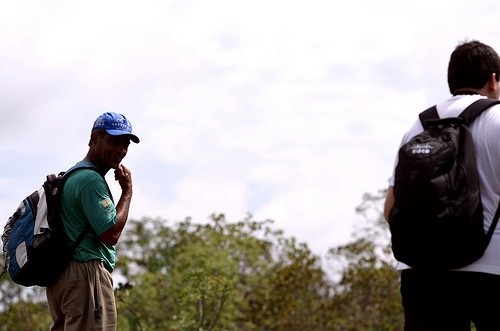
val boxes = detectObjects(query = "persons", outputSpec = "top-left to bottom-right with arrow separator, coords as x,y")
381,38 -> 500,331
38,110 -> 140,331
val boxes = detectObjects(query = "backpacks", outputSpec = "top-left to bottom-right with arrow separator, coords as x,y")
387,97 -> 500,271
0,163 -> 103,290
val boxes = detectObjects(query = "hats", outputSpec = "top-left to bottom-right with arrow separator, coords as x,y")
93,112 -> 140,143
